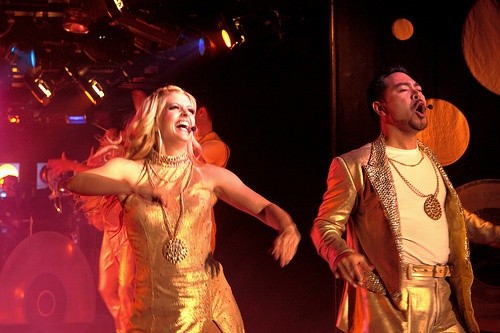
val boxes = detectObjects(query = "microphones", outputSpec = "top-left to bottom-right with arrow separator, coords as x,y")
428,105 -> 434,109
190,126 -> 196,131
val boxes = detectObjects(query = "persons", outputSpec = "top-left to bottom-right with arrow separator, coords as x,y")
311,66 -> 500,333
64,86 -> 300,333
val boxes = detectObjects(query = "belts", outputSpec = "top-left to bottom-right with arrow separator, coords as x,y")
403,263 -> 451,280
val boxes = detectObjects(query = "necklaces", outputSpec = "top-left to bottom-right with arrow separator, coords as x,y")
146,149 -> 193,264
388,148 -> 442,219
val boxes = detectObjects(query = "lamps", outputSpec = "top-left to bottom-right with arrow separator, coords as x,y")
222,17 -> 247,49
85,61 -> 141,105
27,50 -> 96,107
